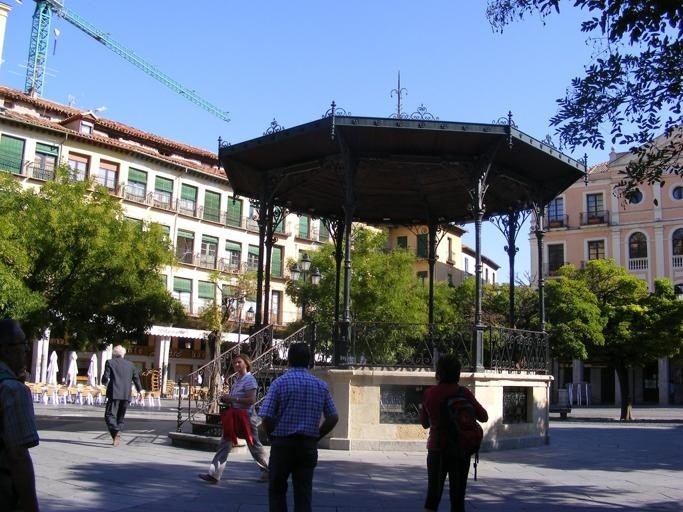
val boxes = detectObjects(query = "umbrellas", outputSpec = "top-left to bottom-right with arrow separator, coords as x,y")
64,351 -> 78,386
85,353 -> 98,386
46,350 -> 59,386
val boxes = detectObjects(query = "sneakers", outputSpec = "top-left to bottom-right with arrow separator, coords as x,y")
111,431 -> 121,446
257,472 -> 268,483
198,473 -> 218,484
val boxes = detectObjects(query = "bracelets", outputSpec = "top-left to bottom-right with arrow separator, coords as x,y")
236,397 -> 239,404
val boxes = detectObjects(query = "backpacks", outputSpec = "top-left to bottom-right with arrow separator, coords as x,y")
440,386 -> 483,460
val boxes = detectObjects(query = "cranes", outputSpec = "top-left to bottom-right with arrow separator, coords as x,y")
25,0 -> 232,123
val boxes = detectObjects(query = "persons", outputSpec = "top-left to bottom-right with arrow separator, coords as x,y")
417,354 -> 489,511
256,342 -> 338,512
196,352 -> 271,483
99,342 -> 146,448
0,310 -> 43,511
